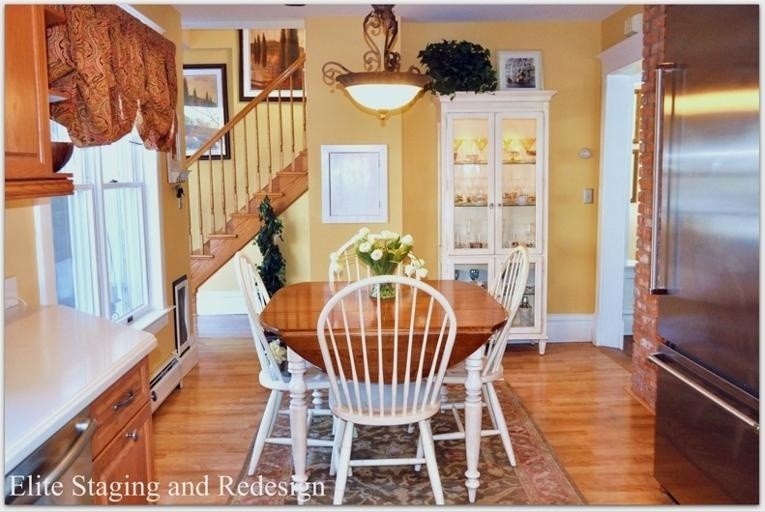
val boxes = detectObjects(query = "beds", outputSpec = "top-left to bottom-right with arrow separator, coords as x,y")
259,279 -> 512,503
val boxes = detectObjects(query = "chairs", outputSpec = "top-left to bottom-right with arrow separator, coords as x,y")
413,245 -> 531,472
314,272 -> 458,504
232,249 -> 354,476
330,233 -> 423,429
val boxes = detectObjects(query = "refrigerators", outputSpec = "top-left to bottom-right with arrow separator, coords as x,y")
647,4 -> 760,504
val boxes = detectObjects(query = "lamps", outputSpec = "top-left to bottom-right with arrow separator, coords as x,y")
322,5 -> 435,121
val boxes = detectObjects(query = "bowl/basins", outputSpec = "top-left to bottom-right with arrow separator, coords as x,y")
49,142 -> 73,172
470,243 -> 483,248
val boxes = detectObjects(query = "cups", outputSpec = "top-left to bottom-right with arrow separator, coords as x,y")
519,305 -> 532,327
470,269 -> 479,280
518,195 -> 528,205
455,269 -> 459,280
525,285 -> 535,294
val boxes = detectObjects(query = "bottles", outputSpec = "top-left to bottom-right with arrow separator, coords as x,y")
522,296 -> 529,307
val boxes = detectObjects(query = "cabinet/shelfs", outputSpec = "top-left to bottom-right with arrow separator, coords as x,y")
5,3 -> 72,204
90,354 -> 157,505
430,88 -> 555,355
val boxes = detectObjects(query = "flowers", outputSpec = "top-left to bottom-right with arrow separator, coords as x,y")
355,227 -> 415,296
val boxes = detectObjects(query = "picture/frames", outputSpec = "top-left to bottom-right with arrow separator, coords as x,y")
494,48 -> 543,89
182,62 -> 231,160
319,143 -> 388,226
236,29 -> 306,103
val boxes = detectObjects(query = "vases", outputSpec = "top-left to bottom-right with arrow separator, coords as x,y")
368,264 -> 398,298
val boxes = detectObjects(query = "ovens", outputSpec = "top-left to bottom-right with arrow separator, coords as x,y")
3,404 -> 97,504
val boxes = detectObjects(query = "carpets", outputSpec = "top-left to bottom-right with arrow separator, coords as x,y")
224,376 -> 591,505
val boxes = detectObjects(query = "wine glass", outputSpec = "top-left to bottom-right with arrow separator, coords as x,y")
454,137 -> 488,164
499,136 -> 537,163
